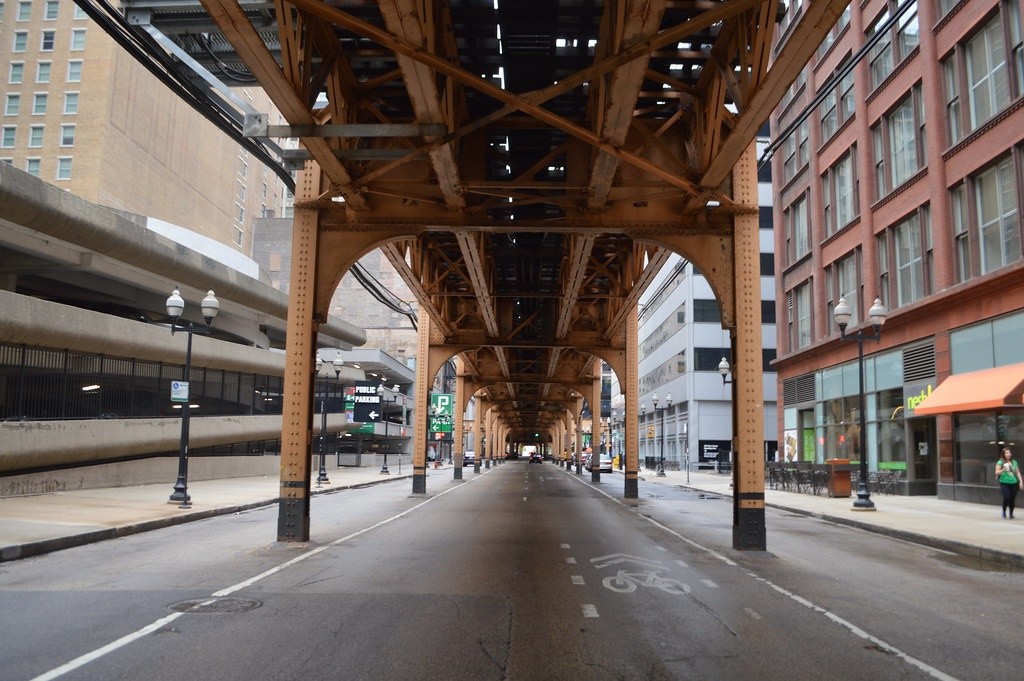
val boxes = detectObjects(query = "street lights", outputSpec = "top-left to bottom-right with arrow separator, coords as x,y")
314,348 -> 343,486
376,382 -> 400,476
166,285 -> 221,505
833,292 -> 889,509
581,410 -> 625,472
652,390 -> 674,477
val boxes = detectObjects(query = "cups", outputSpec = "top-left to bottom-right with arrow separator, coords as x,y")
1004,463 -> 1009,472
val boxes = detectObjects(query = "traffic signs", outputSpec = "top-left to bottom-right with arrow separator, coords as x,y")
353,381 -> 384,423
430,394 -> 453,418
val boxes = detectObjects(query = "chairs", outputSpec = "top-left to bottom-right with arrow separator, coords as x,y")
769,467 -> 896,496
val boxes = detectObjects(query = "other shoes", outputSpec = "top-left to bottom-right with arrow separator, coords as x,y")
1010,516 -> 1014,520
1002,511 -> 1006,518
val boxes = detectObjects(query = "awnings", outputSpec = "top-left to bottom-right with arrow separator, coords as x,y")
914,362 -> 1024,416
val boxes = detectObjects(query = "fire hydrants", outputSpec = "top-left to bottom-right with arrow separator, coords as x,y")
434,460 -> 438,470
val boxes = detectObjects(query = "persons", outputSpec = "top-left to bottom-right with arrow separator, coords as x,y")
995,447 -> 1024,520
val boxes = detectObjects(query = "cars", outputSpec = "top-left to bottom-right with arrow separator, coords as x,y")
563,452 -> 592,469
528,453 -> 544,464
587,454 -> 612,472
463,451 -> 483,467
